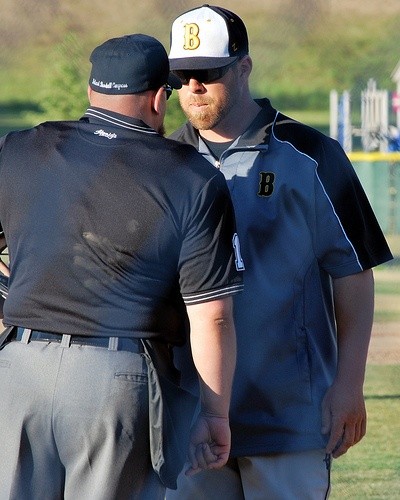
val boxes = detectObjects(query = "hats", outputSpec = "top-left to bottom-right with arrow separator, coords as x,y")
88,34 -> 182,95
168,3 -> 249,70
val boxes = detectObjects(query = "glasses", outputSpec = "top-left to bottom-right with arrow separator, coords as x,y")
171,58 -> 239,85
158,83 -> 172,100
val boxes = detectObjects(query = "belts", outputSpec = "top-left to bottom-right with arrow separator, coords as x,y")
13,326 -> 150,355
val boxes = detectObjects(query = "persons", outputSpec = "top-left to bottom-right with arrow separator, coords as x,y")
0,33 -> 245,500
165,4 -> 393,500
